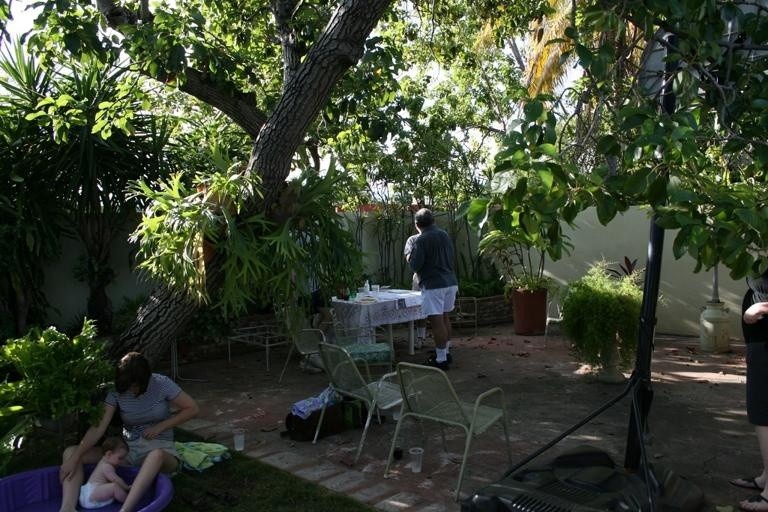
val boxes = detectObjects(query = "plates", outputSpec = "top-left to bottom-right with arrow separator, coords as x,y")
359,297 -> 376,303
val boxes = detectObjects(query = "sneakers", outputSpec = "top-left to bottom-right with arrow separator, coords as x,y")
422,353 -> 452,371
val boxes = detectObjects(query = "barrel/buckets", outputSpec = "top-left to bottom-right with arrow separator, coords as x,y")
511,284 -> 548,337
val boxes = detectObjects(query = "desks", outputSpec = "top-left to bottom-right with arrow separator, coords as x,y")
333,288 -> 419,361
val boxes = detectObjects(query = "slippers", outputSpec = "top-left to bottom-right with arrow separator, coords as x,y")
730,477 -> 767,511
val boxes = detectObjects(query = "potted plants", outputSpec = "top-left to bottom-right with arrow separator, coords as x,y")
553,261 -> 649,384
466,105 -> 590,335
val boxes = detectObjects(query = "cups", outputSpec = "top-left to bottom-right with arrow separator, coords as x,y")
232,428 -> 245,451
371,285 -> 379,297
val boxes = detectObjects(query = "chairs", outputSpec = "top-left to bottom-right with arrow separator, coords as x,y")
311,342 -> 422,467
384,362 -> 511,503
279,309 -> 346,389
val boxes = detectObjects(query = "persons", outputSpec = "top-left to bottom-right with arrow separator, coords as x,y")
80,435 -> 132,510
403,220 -> 454,351
286,216 -> 334,377
727,203 -> 768,512
51,351 -> 202,512
402,209 -> 458,371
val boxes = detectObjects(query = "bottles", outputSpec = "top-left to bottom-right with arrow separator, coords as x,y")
364,279 -> 369,292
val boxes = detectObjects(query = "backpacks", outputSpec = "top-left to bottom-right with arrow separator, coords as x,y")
340,395 -> 368,429
